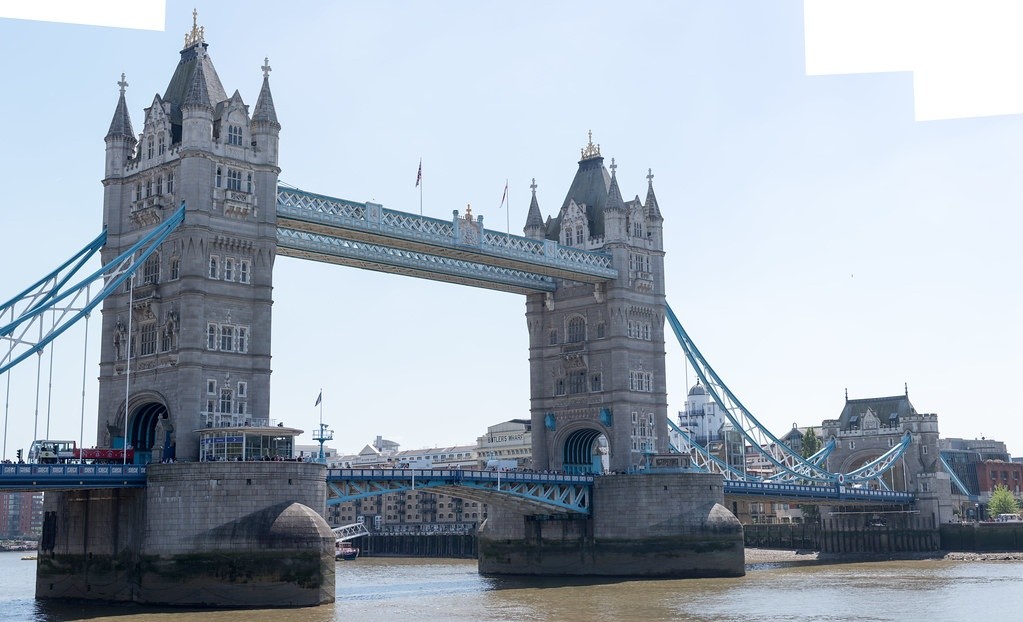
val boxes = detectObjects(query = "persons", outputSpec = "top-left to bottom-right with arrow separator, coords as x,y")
487,465 -> 627,478
947,510 -> 1022,523
1,452 -> 313,465
328,460 -> 460,470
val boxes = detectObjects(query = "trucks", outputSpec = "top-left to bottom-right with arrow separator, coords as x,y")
485,460 -> 518,471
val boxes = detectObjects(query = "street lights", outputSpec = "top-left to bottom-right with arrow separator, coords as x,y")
974,503 -> 979,522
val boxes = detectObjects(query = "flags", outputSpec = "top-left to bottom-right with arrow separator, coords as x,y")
415,161 -> 421,187
314,392 -> 321,406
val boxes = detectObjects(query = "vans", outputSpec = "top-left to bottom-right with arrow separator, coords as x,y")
997,513 -> 1022,523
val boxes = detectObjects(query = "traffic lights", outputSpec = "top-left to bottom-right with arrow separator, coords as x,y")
16,449 -> 21,459
